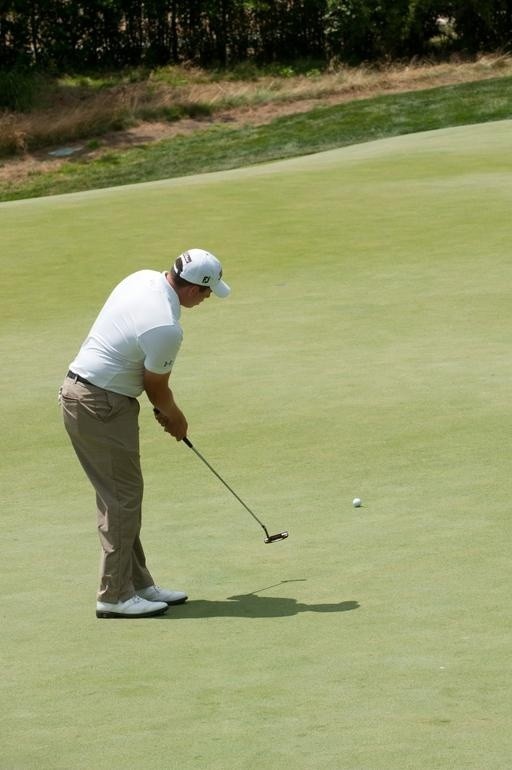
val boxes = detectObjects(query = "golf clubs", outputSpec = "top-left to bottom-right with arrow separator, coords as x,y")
151,409 -> 289,544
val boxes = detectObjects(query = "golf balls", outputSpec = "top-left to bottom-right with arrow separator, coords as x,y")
352,499 -> 361,507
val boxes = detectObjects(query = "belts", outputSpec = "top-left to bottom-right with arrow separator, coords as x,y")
66,370 -> 91,385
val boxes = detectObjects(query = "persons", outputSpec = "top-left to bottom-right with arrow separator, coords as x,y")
58,246 -> 235,620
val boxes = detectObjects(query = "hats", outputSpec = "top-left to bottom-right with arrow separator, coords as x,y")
173,248 -> 231,297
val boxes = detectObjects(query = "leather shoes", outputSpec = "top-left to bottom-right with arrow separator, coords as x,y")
96,594 -> 169,618
136,583 -> 188,605
